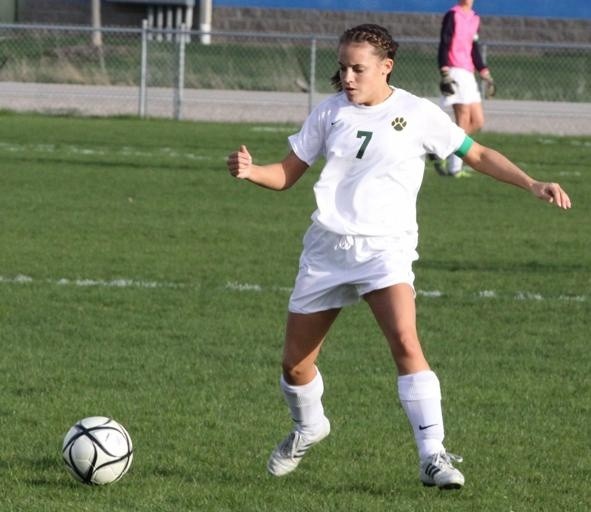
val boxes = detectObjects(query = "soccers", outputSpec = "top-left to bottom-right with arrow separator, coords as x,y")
60,415 -> 134,486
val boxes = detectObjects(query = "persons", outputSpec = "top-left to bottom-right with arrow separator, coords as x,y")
226,23 -> 573,487
439,1 -> 494,135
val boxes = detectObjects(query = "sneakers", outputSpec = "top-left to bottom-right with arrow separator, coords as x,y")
266,416 -> 331,478
434,158 -> 474,178
418,452 -> 465,490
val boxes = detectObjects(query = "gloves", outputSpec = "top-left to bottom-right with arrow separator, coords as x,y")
439,69 -> 460,96
479,73 -> 497,98
